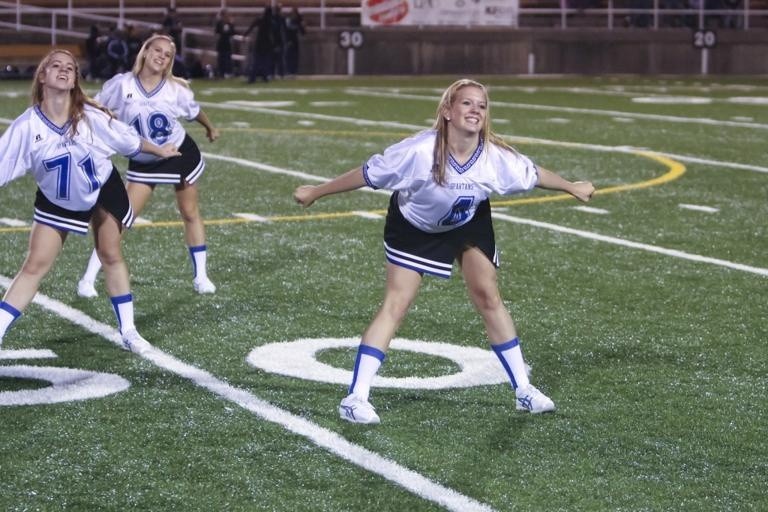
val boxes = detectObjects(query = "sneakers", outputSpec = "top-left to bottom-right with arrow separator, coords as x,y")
515,384 -> 554,413
193,276 -> 216,295
339,395 -> 381,424
77,279 -> 99,298
122,329 -> 151,355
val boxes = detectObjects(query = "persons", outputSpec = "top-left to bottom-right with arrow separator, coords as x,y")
0,48 -> 183,354
78,33 -> 220,299
82,0 -> 306,83
292,79 -> 595,425
